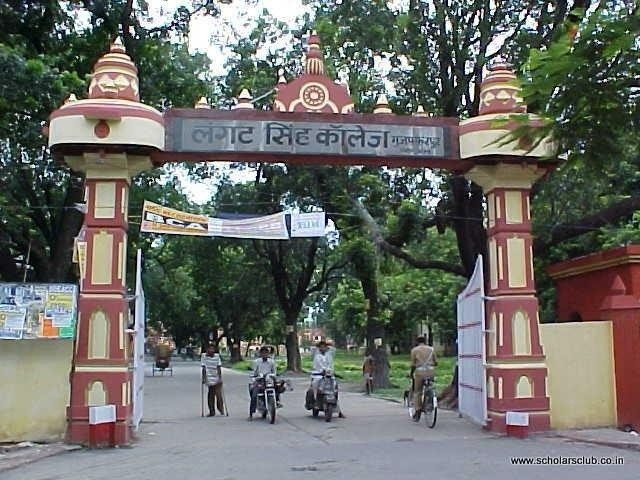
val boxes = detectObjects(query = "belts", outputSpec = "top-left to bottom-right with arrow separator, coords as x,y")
416,366 -> 434,371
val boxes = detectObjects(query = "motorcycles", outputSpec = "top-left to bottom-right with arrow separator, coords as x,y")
246,366 -> 344,424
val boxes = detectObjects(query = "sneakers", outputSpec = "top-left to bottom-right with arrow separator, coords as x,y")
413,409 -> 422,419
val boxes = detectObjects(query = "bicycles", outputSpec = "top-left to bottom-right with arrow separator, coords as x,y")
404,374 -> 438,429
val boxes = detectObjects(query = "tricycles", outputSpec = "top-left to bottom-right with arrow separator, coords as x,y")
152,345 -> 174,377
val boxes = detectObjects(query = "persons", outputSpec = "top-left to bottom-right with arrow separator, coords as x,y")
200,344 -> 225,417
374,342 -> 392,389
310,340 -> 347,418
245,347 -> 283,421
362,351 -> 377,396
407,335 -> 439,424
